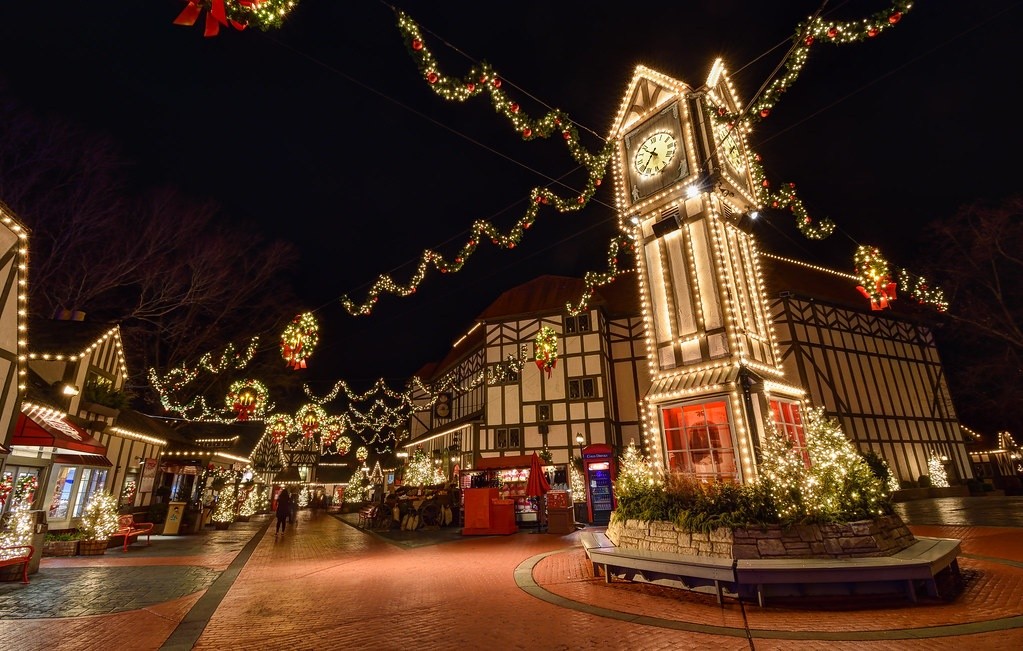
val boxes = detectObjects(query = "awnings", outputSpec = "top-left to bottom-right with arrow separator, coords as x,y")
477,455 -> 545,469
9,404 -> 107,457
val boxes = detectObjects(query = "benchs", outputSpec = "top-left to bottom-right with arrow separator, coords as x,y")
891,540 -> 963,598
579,532 -> 612,579
357,504 -> 379,529
113,516 -> 153,553
0,545 -> 34,584
736,557 -> 930,607
588,546 -> 735,604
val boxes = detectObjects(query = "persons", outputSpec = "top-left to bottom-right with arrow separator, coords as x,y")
275,489 -> 291,536
446,484 -> 461,528
320,494 -> 327,513
308,493 -> 320,520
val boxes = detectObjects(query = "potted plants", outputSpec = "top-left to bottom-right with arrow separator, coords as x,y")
75,488 -> 118,555
211,486 -> 270,530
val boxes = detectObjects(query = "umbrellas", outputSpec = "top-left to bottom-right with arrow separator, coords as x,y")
524,450 -> 553,535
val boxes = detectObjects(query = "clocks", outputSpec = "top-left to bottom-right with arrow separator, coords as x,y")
720,128 -> 746,173
634,131 -> 675,176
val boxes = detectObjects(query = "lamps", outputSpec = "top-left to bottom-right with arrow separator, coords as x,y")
135,456 -> 146,465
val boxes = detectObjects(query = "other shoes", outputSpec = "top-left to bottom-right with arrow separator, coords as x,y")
281,532 -> 284,536
274,533 -> 278,536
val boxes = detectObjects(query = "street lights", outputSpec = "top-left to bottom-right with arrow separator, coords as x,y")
361,467 -> 369,498
397,450 -> 408,483
576,433 -> 584,460
